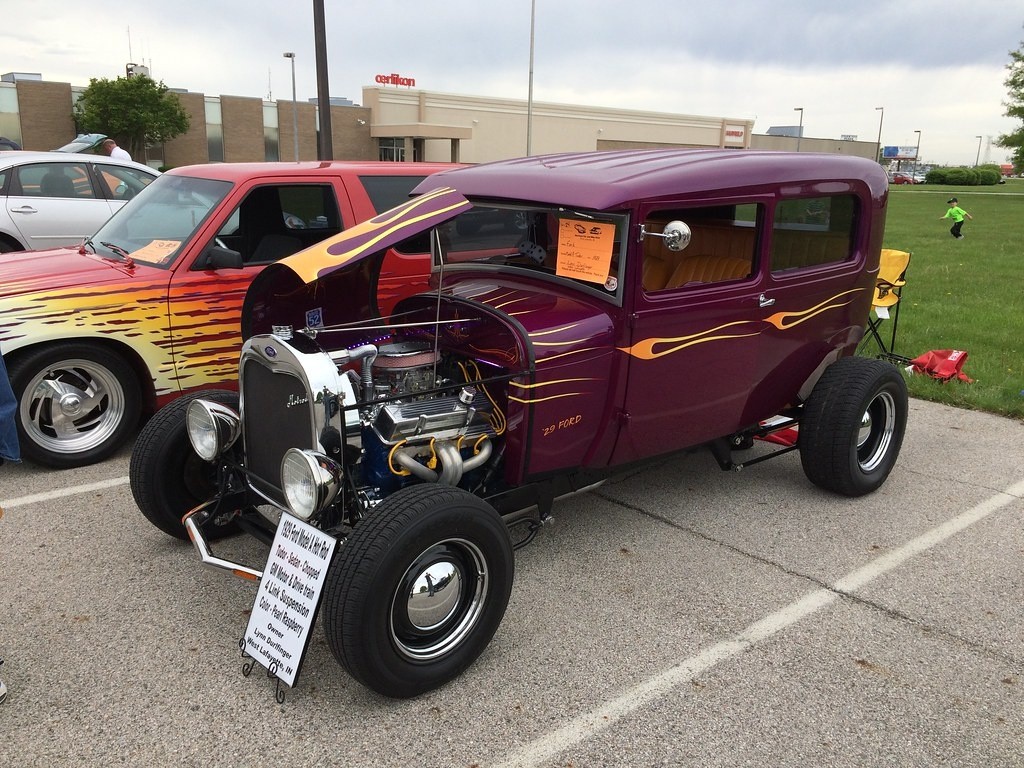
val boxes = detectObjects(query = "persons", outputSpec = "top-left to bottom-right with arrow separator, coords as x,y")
103,139 -> 132,162
40,165 -> 77,198
939,198 -> 973,240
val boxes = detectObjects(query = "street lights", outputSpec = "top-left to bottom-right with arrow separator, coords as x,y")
283,52 -> 298,161
875,107 -> 884,162
975,136 -> 982,166
912,131 -> 921,184
795,107 -> 804,151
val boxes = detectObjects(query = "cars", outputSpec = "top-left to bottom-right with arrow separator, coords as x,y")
0,132 -> 108,153
887,170 -> 927,184
0,158 -> 481,470
129,143 -> 915,701
0,149 -> 162,252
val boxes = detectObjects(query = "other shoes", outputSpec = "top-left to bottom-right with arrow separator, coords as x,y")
958,235 -> 964,239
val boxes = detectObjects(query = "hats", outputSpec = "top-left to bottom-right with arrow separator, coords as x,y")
947,198 -> 957,203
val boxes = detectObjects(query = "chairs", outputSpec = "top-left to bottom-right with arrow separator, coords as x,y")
878,348 -> 973,383
40,171 -> 76,197
247,235 -> 303,264
854,249 -> 911,357
640,255 -> 753,290
